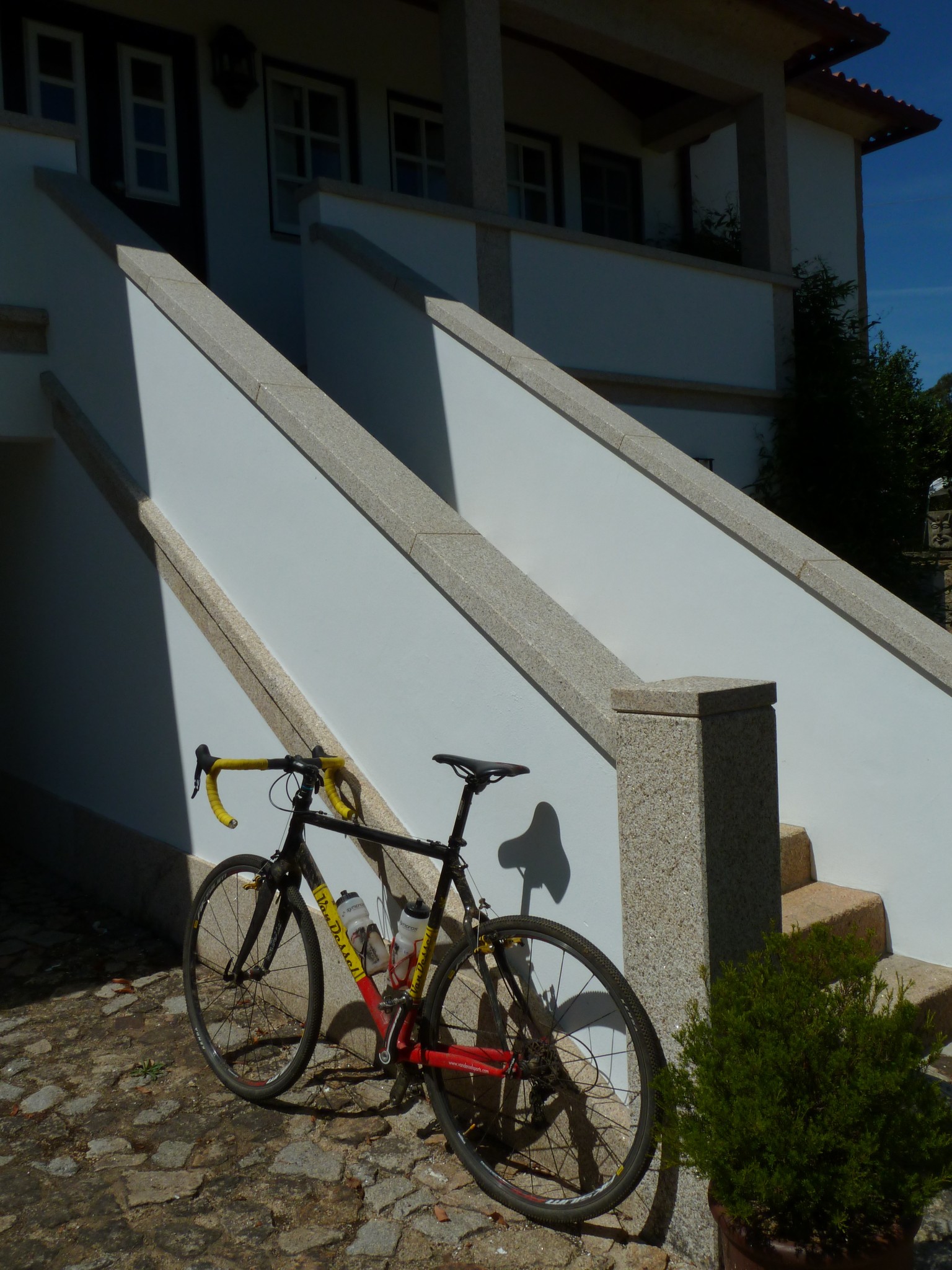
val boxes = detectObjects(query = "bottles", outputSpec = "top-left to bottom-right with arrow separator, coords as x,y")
336,890 -> 390,975
385,898 -> 430,991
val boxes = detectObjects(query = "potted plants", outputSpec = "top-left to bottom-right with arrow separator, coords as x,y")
641,913 -> 952,1270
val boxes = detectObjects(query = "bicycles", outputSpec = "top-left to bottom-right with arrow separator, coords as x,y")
181,743 -> 667,1229
